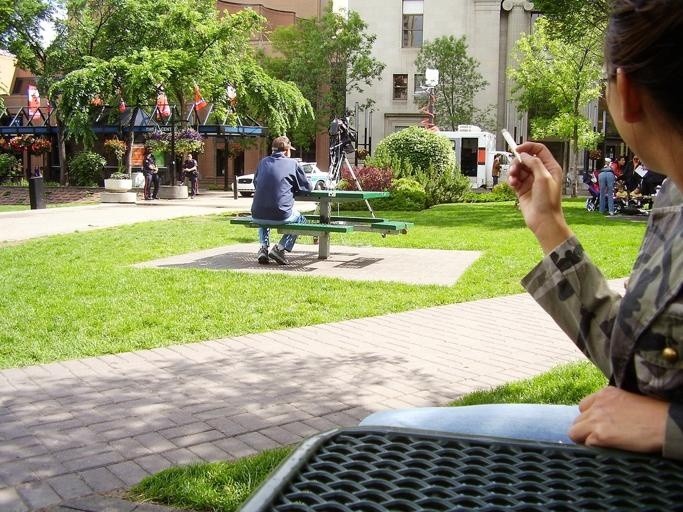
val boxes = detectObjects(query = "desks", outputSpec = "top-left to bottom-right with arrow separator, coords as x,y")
294,187 -> 390,259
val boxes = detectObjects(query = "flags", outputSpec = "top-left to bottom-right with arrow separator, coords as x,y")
225,85 -> 237,106
114,87 -> 125,113
27,83 -> 41,118
156,84 -> 172,119
193,80 -> 207,111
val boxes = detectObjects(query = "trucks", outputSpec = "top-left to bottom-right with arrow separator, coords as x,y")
439,122 -> 516,190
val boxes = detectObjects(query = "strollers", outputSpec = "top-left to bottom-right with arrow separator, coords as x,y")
578,169 -> 624,214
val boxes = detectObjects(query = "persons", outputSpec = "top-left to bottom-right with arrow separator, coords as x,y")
594,154 -> 664,217
179,154 -> 198,199
143,151 -> 160,200
508,0 -> 682,460
492,153 -> 501,186
250,137 -> 312,265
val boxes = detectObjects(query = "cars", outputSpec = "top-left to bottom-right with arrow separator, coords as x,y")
230,161 -> 336,198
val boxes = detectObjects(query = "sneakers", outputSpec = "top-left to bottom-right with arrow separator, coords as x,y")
145,196 -> 160,200
609,211 -> 615,216
269,244 -> 289,265
257,247 -> 268,264
191,195 -> 195,199
179,182 -> 184,186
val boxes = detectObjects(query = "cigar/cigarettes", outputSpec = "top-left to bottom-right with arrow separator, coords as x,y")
499,126 -> 522,163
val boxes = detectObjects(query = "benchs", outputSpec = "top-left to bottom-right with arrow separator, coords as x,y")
230,214 -> 355,259
300,214 -> 415,258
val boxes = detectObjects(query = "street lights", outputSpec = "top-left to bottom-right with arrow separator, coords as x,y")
423,67 -> 439,128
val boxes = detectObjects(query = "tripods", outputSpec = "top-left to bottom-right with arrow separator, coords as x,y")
313,151 -> 375,218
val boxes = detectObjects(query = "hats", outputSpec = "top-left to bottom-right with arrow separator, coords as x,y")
272,136 -> 296,151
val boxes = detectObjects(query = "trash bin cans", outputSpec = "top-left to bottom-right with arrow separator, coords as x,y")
29,177 -> 45,209
